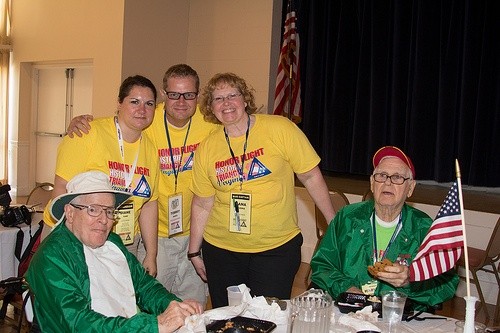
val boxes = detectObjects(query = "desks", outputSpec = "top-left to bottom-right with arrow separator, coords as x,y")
0,204 -> 44,285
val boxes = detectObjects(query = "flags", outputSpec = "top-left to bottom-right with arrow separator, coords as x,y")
409,180 -> 464,282
274,0 -> 302,124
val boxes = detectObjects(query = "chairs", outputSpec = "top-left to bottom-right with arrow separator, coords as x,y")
455,217 -> 500,320
26,183 -> 55,208
0,277 -> 36,333
306,188 -> 350,280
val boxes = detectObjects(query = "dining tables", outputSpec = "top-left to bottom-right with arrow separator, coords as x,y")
171,300 -> 495,333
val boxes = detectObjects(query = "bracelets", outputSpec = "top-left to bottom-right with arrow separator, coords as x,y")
188,251 -> 201,258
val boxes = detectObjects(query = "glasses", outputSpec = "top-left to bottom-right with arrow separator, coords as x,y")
165,89 -> 198,100
71,204 -> 119,219
374,173 -> 412,185
213,93 -> 243,102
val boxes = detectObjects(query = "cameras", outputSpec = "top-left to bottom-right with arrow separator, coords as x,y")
0,184 -> 32,227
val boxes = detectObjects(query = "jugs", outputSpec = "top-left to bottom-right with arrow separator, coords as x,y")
287,288 -> 332,333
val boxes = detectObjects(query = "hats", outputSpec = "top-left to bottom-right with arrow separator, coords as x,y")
373,146 -> 415,177
49,171 -> 133,222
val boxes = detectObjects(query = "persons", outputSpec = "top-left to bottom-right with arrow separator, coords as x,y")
310,146 -> 460,312
23,170 -> 204,333
189,73 -> 336,309
40,75 -> 161,278
66,64 -> 222,306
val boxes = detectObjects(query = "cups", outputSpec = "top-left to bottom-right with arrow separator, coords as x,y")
382,291 -> 407,324
227,286 -> 250,307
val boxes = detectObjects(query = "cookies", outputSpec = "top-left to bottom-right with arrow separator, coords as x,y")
368,258 -> 392,277
